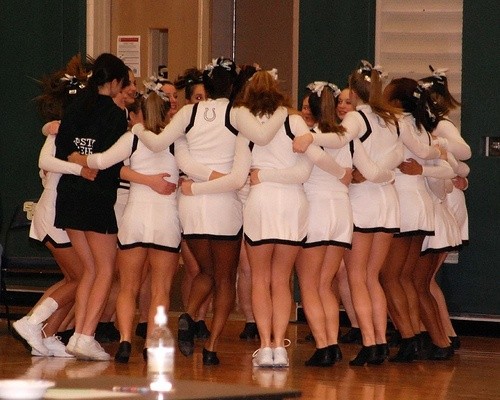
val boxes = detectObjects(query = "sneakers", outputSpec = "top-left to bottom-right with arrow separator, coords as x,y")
11,316 -> 49,356
272,339 -> 292,368
31,334 -> 76,358
251,346 -> 273,368
65,333 -> 111,361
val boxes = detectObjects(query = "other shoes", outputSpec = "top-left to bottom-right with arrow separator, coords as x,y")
114,340 -> 131,364
239,321 -> 259,340
429,343 -> 455,360
196,320 -> 211,340
340,326 -> 362,344
387,329 -> 402,347
96,321 -> 122,342
448,334 -> 461,348
349,344 -> 389,367
177,313 -> 194,357
389,334 -> 426,363
135,322 -> 147,337
304,343 -> 343,367
202,349 -> 220,365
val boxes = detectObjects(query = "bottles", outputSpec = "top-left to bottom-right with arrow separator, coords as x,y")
145,305 -> 176,392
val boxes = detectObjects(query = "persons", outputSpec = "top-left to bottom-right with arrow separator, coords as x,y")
11,52 -> 471,366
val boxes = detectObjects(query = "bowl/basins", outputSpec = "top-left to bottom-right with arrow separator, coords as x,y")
0,378 -> 55,400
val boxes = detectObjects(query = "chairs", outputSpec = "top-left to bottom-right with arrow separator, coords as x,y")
0,205 -> 21,332
